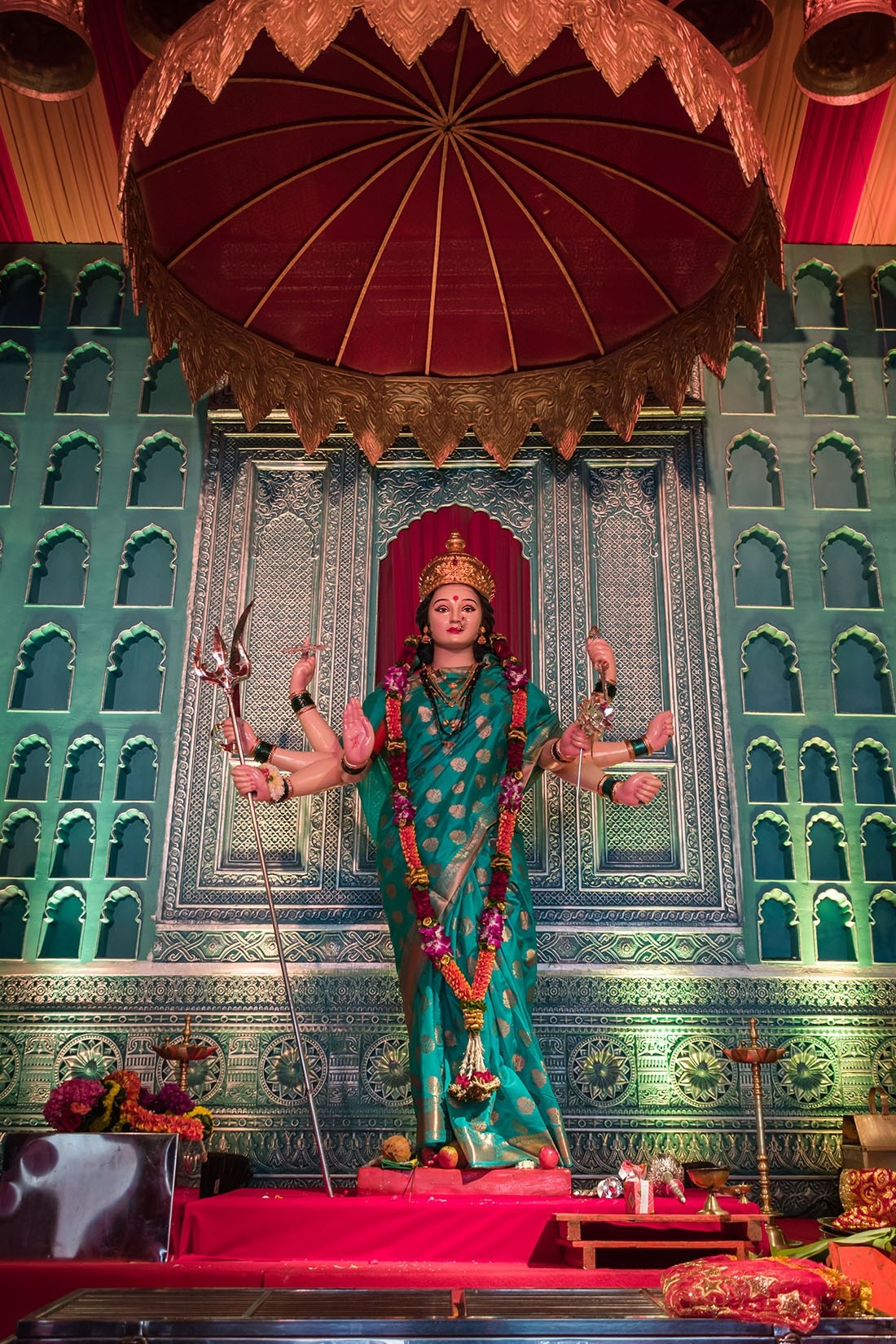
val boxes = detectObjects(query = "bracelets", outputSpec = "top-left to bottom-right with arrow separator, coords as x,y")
247,737 -> 278,764
341,753 -> 369,775
597,774 -> 623,806
287,689 -> 316,717
269,778 -> 294,804
624,734 -> 654,762
550,737 -> 575,766
591,678 -> 619,703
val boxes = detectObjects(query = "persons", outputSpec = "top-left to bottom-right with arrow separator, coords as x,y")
221,532 -> 674,1167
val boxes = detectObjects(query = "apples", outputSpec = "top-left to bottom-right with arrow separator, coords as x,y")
539,1146 -> 558,1170
437,1146 -> 459,1170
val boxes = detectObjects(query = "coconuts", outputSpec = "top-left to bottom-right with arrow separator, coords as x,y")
382,1134 -> 410,1162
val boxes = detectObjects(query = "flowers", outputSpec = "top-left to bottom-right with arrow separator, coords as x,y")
41,1068 -> 207,1141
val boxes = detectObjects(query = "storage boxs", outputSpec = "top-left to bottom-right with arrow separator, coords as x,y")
626,1180 -> 655,1214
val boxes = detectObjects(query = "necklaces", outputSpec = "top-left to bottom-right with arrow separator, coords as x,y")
418,661 -> 483,754
